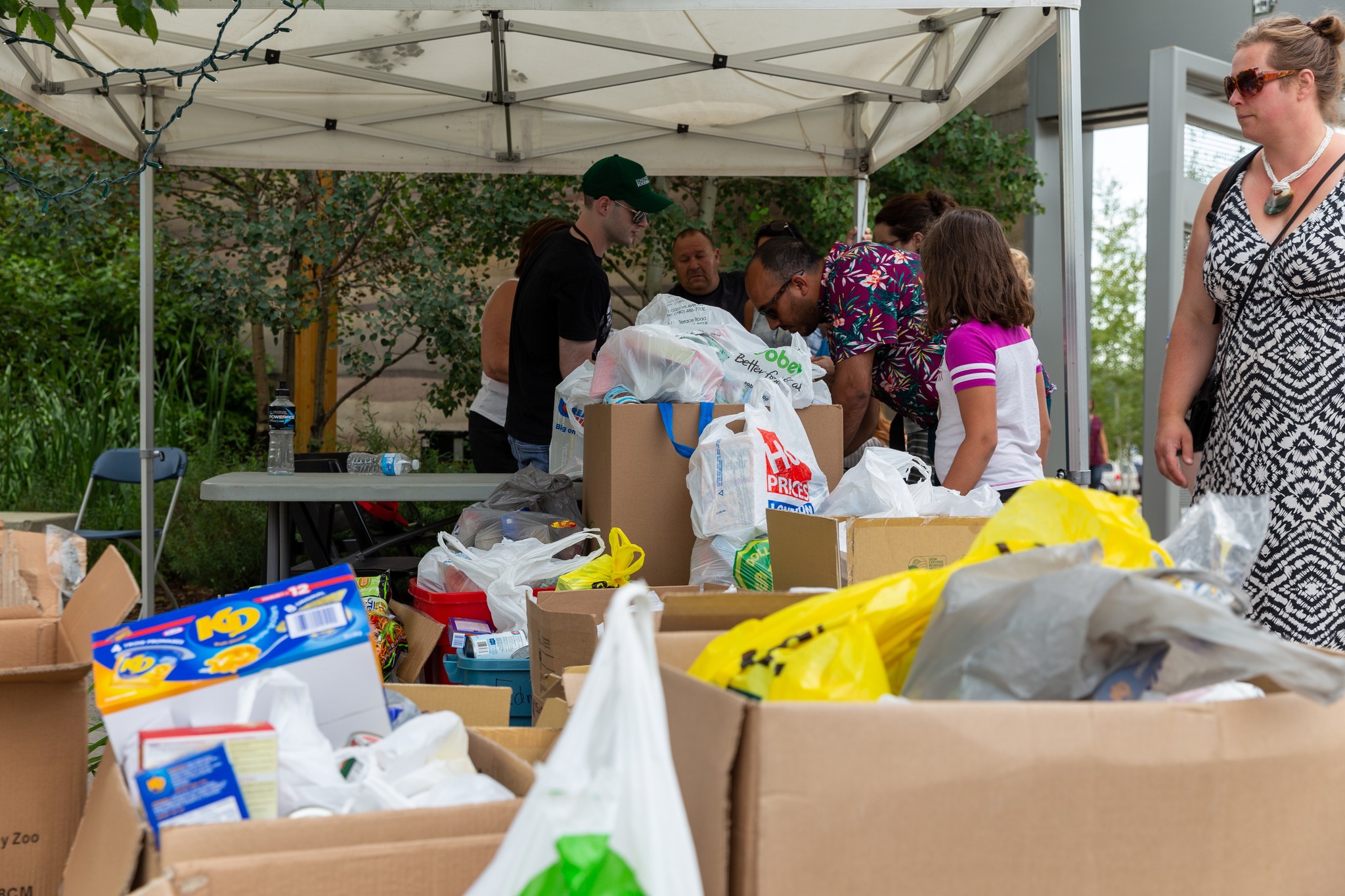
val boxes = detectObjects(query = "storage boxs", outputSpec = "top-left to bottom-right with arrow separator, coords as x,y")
0,403 -> 1345,896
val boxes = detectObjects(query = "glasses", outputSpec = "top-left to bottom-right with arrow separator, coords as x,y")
759,270 -> 804,319
593,197 -> 648,225
1223,67 -> 1299,101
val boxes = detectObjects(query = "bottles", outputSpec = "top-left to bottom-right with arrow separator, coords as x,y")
346,452 -> 420,476
267,381 -> 295,476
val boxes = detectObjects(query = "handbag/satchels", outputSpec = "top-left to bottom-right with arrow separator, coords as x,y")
1175,373 -> 1221,456
467,577 -> 708,896
413,288 -> 1345,685
230,672 -> 413,818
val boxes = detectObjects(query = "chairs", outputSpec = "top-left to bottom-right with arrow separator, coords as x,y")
72,446 -> 187,626
279,448 -> 461,595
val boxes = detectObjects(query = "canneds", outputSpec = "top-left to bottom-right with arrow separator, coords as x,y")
602,387 -> 642,404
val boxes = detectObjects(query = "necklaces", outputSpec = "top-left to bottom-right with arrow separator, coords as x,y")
1261,122 -> 1333,215
571,224 -> 599,264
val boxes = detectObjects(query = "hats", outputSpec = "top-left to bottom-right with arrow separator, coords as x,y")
583,154 -> 674,213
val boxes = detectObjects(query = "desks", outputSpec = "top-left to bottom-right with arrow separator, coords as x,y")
201,471 -> 584,600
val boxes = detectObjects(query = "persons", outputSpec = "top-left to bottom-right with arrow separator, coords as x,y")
1155,13 -> 1345,648
468,154 -> 1110,503
